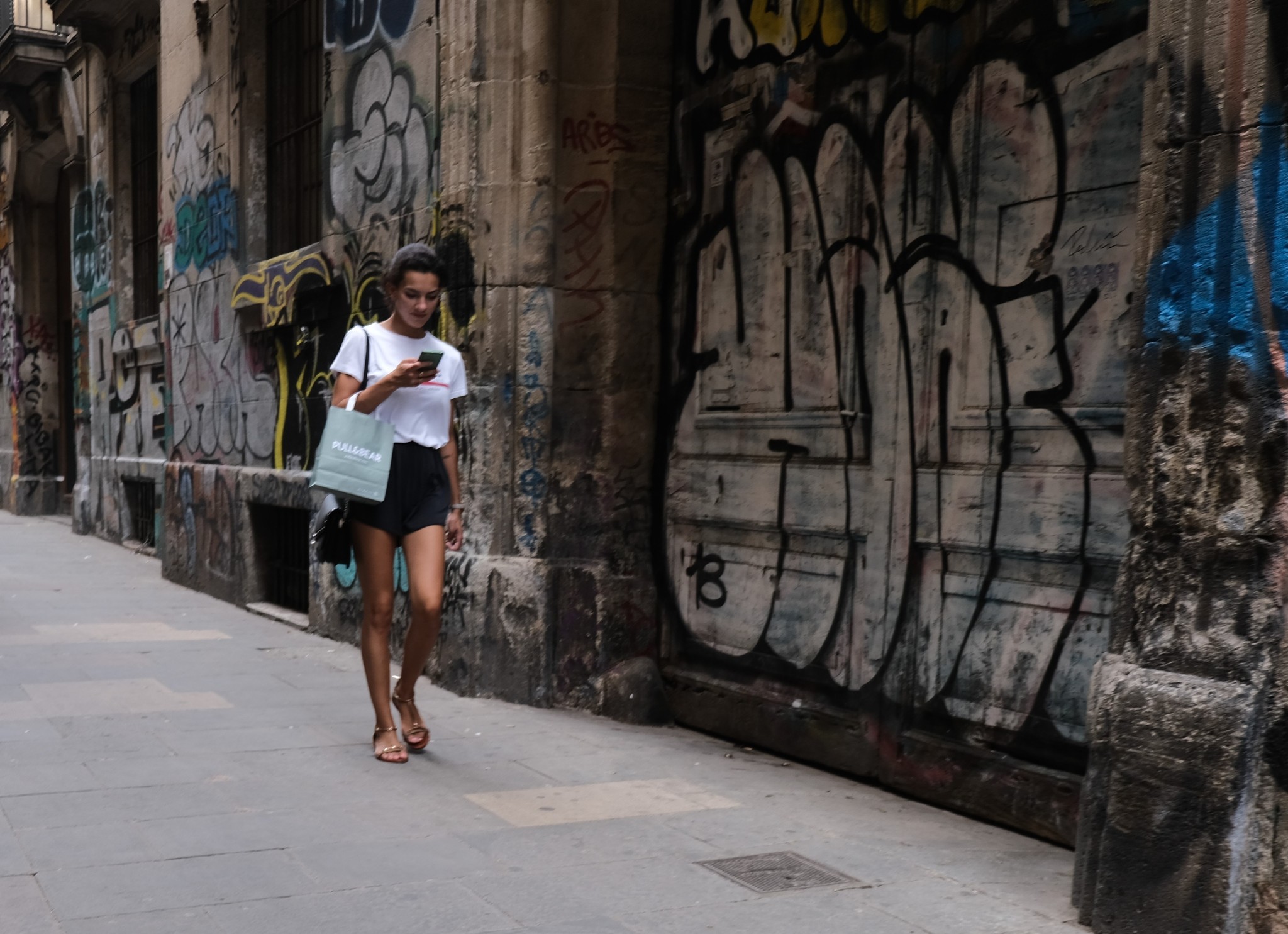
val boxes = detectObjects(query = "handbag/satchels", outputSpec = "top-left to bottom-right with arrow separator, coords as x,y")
309,492 -> 352,568
308,390 -> 395,501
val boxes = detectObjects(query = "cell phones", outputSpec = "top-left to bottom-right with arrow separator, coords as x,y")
416,349 -> 444,378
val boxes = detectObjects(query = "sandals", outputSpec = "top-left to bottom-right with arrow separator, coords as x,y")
391,677 -> 429,749
372,723 -> 409,763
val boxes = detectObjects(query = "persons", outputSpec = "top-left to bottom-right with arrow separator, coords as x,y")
327,242 -> 470,764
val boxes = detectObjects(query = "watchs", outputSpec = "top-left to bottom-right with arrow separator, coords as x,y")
449,502 -> 465,513
